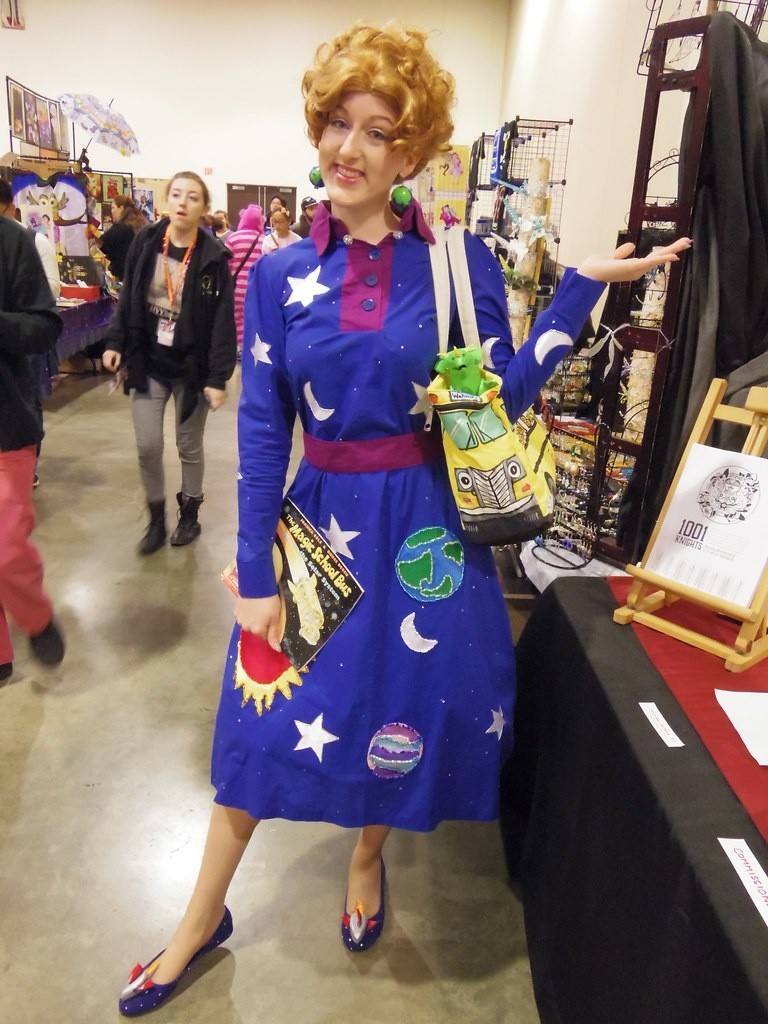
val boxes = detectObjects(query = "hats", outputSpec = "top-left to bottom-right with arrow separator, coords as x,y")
302,197 -> 318,209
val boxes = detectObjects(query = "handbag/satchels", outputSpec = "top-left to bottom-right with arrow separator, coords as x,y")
420,224 -> 558,544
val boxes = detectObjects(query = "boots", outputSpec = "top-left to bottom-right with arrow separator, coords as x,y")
171,492 -> 204,547
139,499 -> 166,553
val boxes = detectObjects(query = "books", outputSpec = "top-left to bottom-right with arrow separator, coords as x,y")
220,496 -> 365,672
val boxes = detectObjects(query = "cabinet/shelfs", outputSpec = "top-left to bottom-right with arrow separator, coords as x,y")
415,115 -> 574,344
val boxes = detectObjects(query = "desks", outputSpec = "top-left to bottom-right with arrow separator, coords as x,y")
496,574 -> 768,1024
41,296 -> 116,404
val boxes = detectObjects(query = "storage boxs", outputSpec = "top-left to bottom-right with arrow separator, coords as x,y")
57,347 -> 87,374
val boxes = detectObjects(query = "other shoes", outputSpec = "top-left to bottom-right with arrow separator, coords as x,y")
118,903 -> 233,1015
30,621 -> 63,666
0,662 -> 13,682
343,846 -> 386,953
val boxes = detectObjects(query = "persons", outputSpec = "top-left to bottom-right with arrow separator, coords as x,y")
99,195 -> 150,280
208,195 -> 318,364
0,182 -> 65,683
102,172 -> 238,555
119,26 -> 692,1018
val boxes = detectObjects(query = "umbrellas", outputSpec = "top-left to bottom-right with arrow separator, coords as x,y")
59,93 -> 140,159
